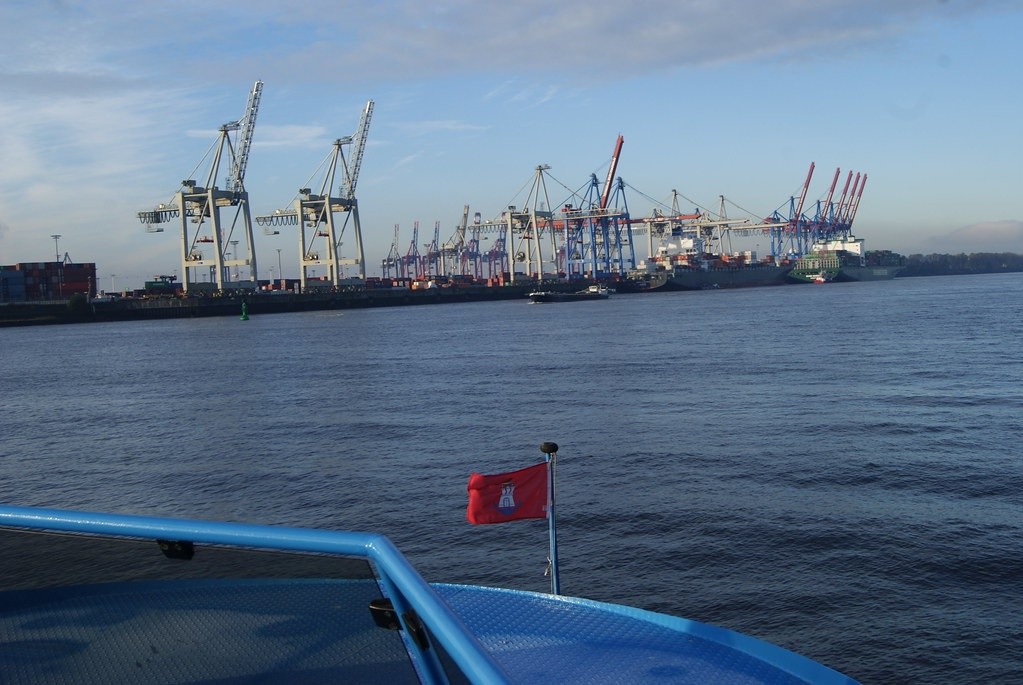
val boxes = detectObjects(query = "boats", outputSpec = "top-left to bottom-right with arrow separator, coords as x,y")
785,252 -> 839,284
529,282 -> 616,302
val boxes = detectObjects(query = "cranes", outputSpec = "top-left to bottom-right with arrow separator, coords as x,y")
771,161 -> 867,262
382,204 -> 503,279
558,133 -> 635,282
252,97 -> 375,294
136,78 -> 265,295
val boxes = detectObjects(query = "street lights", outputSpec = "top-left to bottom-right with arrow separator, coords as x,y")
225,252 -> 231,282
50,233 -> 63,299
230,240 -> 239,274
338,241 -> 344,273
276,248 -> 282,280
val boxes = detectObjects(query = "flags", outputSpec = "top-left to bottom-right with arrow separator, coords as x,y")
465,460 -> 550,524
